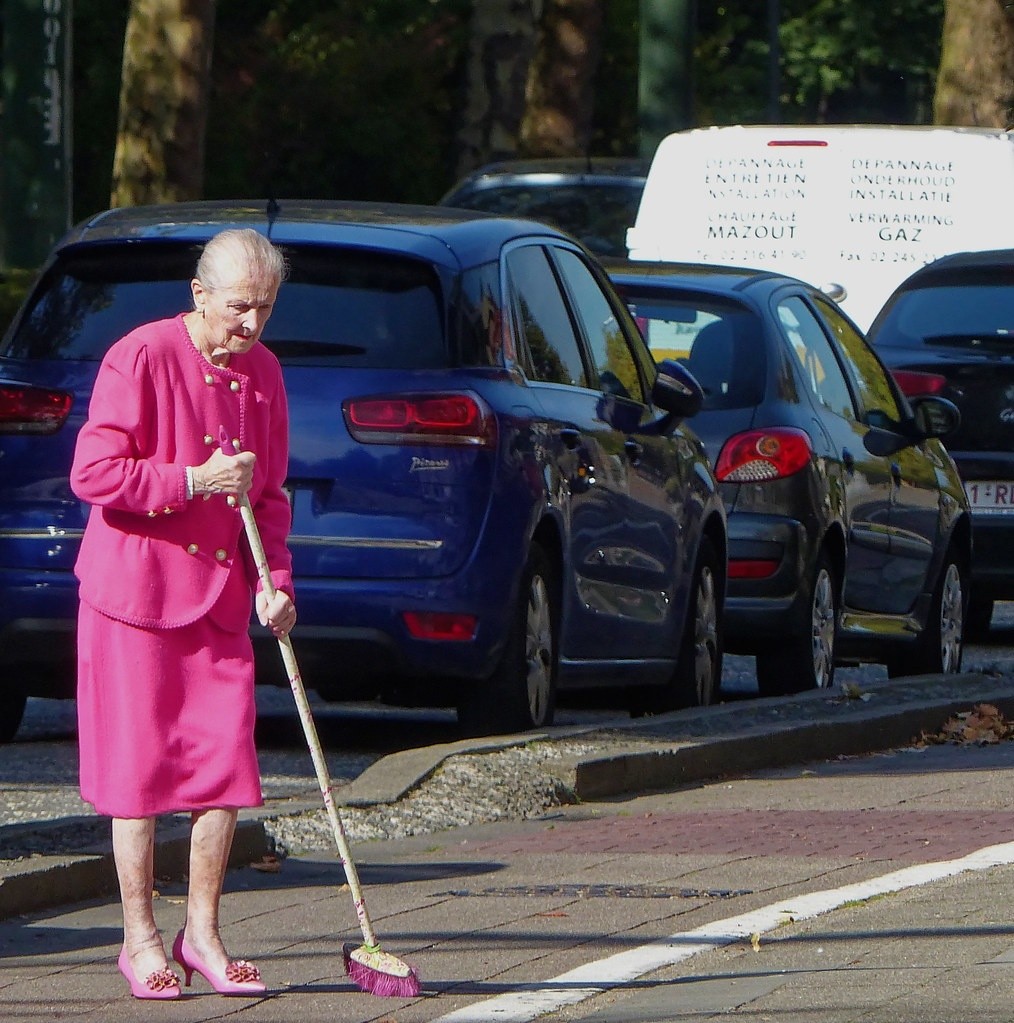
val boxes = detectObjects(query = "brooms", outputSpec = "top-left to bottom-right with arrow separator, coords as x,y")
217,425 -> 421,997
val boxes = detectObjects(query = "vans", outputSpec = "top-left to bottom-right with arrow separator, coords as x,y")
627,126 -> 1014,410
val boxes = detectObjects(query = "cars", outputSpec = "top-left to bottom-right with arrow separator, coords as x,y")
597,260 -> 975,698
438,155 -> 657,259
2,194 -> 730,739
865,246 -> 1013,649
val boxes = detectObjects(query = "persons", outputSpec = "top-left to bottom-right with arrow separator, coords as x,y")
70,226 -> 297,1000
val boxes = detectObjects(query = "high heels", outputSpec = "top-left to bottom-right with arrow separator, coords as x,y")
118,943 -> 181,1000
171,929 -> 267,996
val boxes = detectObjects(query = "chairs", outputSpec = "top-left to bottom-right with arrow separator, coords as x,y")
689,321 -> 735,408
